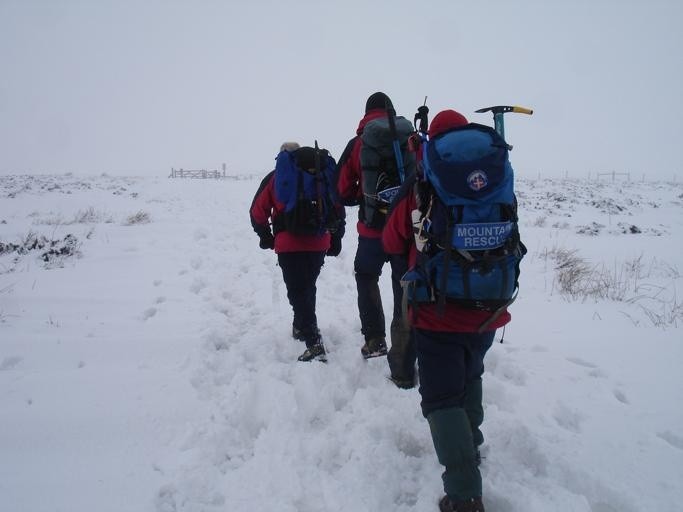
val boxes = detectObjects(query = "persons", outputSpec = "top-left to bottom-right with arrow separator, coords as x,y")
336,91 -> 419,389
249,142 -> 346,361
382,110 -> 526,512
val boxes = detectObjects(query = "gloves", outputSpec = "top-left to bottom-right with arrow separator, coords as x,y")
253,221 -> 274,250
327,234 -> 342,256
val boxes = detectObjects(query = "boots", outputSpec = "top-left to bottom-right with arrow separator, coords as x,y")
293,326 -> 328,362
439,495 -> 485,512
361,337 -> 388,359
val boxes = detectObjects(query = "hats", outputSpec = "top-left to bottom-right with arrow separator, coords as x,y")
366,92 -> 396,114
429,109 -> 468,140
275,142 -> 300,160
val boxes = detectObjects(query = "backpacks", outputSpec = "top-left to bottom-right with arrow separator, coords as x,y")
400,123 -> 527,335
358,116 -> 415,232
275,146 -> 337,236
